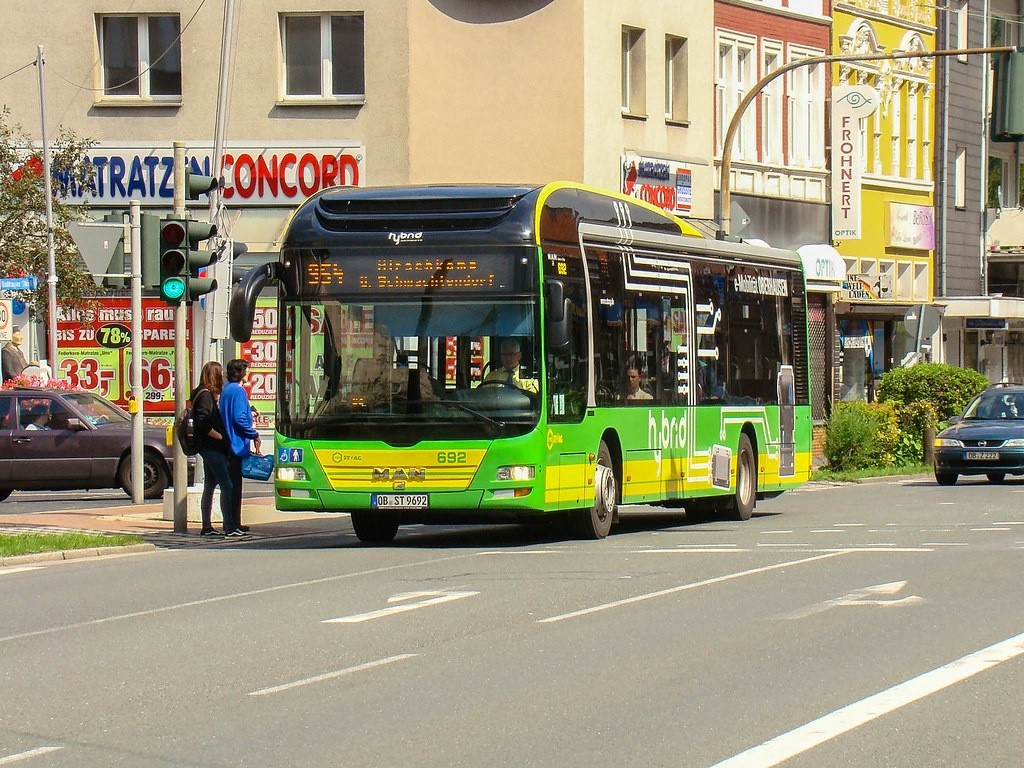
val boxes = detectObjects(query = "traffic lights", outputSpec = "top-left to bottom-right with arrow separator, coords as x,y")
186,219 -> 217,306
186,167 -> 218,200
213,238 -> 248,343
159,214 -> 189,303
101,210 -> 129,290
140,212 -> 161,292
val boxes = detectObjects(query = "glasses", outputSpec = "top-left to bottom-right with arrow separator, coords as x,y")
498,351 -> 519,357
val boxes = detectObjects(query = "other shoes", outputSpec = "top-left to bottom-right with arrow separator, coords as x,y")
239,526 -> 250,532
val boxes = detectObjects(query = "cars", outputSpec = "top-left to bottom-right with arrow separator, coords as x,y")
0,389 -> 195,502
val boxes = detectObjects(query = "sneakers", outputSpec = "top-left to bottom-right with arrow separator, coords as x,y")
224,528 -> 252,539
200,527 -> 221,538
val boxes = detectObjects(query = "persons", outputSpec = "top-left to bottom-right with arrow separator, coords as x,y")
1,324 -> 28,382
25,403 -> 54,431
219,359 -> 262,533
49,401 -> 86,430
483,338 -> 539,394
189,362 -> 252,539
620,358 -> 653,400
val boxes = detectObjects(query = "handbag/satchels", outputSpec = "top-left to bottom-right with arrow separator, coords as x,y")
241,440 -> 274,481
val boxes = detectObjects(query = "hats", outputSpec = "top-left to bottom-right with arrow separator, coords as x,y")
12,325 -> 20,332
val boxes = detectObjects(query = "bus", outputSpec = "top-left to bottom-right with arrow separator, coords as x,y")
228,181 -> 814,544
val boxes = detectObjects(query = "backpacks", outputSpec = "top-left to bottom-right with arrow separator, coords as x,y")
176,389 -> 213,456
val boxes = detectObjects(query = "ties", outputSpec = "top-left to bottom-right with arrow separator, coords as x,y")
505,371 -> 515,388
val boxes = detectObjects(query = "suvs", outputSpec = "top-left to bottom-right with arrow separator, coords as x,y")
933,382 -> 1024,485
